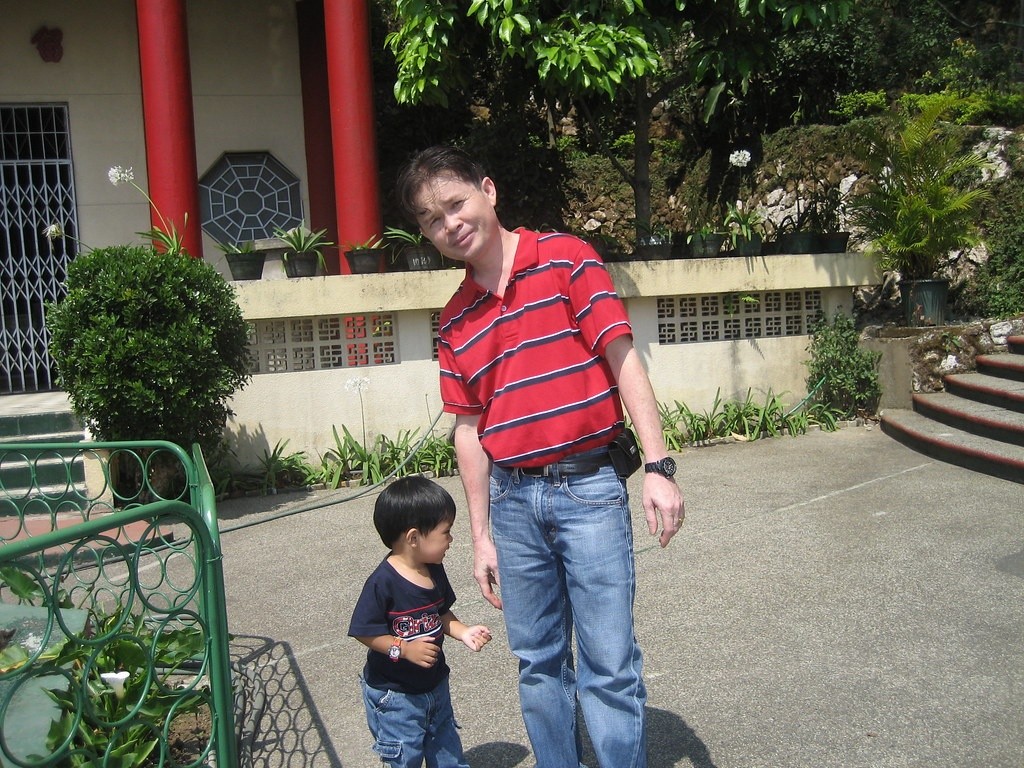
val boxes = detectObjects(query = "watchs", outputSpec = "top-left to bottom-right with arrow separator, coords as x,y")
645,457 -> 677,479
388,637 -> 404,663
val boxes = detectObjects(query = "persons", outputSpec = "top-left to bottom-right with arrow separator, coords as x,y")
396,146 -> 685,768
349,475 -> 492,768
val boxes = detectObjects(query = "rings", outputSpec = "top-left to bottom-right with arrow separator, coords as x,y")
673,517 -> 684,527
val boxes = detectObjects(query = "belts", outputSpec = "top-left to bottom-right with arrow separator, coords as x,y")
495,451 -> 611,477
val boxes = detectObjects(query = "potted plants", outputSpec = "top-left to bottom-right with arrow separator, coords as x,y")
838,95 -> 1007,324
212,238 -> 270,280
797,304 -> 884,416
271,218 -> 333,279
574,149 -> 849,263
332,234 -> 390,274
42,246 -> 260,507
383,226 -> 445,271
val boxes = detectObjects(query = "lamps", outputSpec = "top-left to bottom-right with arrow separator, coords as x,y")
30,26 -> 65,63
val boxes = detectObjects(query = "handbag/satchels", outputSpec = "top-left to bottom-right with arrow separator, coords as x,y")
607,428 -> 643,479
